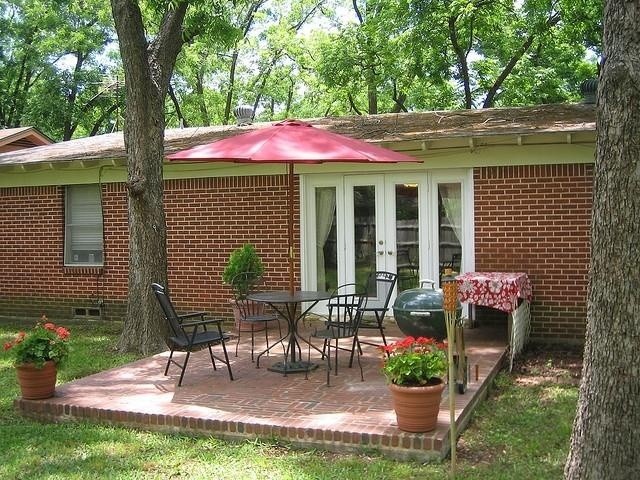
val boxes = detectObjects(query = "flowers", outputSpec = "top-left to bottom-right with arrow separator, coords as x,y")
376,336 -> 449,385
4,315 -> 70,369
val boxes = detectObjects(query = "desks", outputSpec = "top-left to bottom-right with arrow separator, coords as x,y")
455,272 -> 533,347
245,291 -> 336,377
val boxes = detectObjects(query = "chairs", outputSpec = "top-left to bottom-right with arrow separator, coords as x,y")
322,271 -> 398,362
396,248 -> 462,287
231,271 -> 286,363
152,283 -> 234,386
305,284 -> 368,386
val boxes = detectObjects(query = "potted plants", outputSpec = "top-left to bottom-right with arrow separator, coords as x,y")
221,245 -> 268,332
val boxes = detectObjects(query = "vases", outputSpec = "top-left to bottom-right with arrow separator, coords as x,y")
390,377 -> 445,432
16,361 -> 57,400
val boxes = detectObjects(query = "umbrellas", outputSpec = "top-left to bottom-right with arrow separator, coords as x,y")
162,116 -> 426,297
